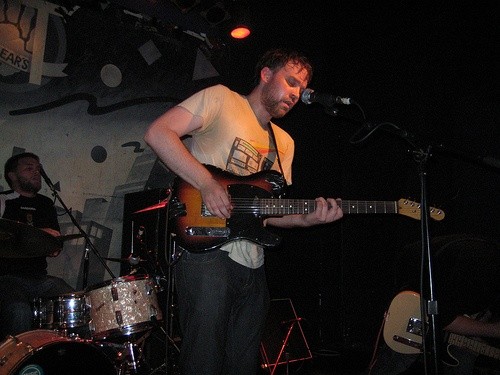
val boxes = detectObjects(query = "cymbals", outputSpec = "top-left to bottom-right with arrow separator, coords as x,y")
103,257 -> 149,265
0,217 -> 60,260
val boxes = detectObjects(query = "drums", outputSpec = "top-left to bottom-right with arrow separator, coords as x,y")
85,273 -> 166,342
0,329 -> 125,375
33,294 -> 90,335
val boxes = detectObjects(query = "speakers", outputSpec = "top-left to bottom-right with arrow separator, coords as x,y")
258,298 -> 312,375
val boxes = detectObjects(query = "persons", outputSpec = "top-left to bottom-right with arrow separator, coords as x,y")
362,234 -> 500,375
0,152 -> 76,337
144,47 -> 344,375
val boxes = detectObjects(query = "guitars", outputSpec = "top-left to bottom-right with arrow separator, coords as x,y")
383,290 -> 500,361
171,163 -> 445,254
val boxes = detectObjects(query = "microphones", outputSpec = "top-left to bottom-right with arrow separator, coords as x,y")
280,317 -> 302,324
302,88 -> 351,105
